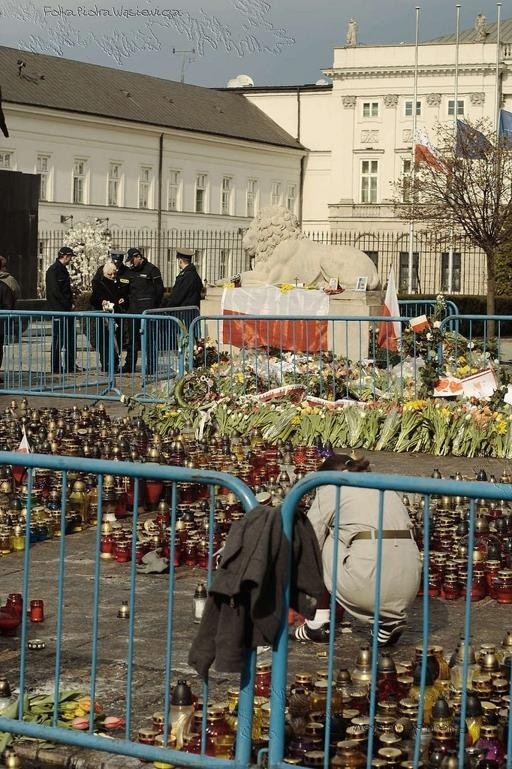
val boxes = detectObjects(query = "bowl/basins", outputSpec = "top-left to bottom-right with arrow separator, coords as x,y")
28,639 -> 45,650
323,287 -> 345,295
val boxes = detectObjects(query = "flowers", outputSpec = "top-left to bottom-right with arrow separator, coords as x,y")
143,395 -> 512,460
139,291 -> 501,395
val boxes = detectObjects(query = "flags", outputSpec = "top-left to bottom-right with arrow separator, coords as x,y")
416,142 -> 448,174
454,116 -> 495,160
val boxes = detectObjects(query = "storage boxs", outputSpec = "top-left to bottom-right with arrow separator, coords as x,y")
455,369 -> 501,399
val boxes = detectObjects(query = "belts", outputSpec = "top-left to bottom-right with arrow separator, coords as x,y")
351,528 -> 416,542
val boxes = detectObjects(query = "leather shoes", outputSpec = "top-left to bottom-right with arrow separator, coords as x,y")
119,365 -> 136,373
50,362 -> 88,375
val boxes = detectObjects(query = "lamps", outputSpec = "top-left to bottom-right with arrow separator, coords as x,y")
94,215 -> 114,240
60,214 -> 76,235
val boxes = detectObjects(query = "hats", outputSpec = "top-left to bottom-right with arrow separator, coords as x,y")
109,249 -> 127,264
58,246 -> 78,258
175,247 -> 196,262
124,247 -> 141,263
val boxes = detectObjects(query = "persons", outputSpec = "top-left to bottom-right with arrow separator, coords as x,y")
46,247 -> 85,374
0,257 -> 21,365
89,239 -> 205,372
288,454 -> 421,648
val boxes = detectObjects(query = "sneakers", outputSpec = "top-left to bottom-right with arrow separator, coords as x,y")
287,618 -> 336,646
369,619 -> 408,649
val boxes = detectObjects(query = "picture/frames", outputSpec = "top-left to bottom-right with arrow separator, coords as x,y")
355,277 -> 369,292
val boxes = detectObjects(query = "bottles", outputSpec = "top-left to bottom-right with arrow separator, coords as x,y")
138,627 -> 511,769
192,581 -> 208,625
397,468 -> 511,605
1,677 -> 11,710
117,601 -> 129,619
7,593 -> 23,623
0,395 -> 317,567
30,599 -> 45,622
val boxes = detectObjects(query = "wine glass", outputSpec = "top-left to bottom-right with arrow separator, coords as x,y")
0,606 -> 21,638
